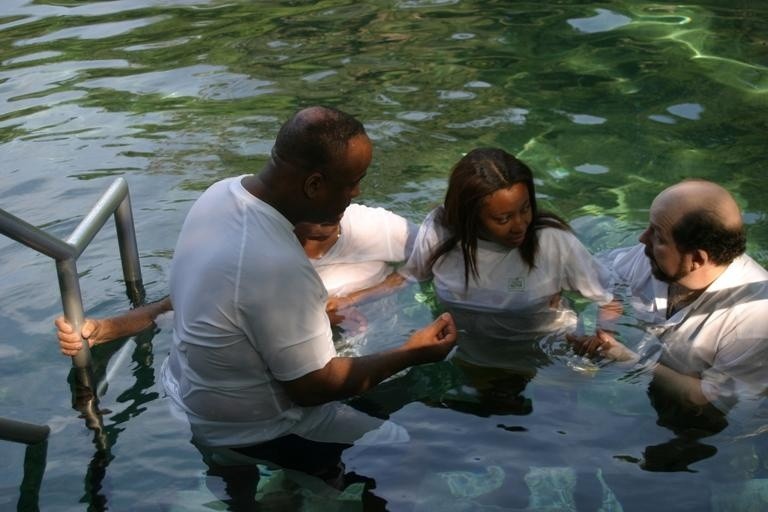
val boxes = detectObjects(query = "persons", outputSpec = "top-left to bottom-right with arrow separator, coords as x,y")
159,104 -> 458,512
564,176 -> 768,416
54,203 -> 423,359
324,146 -> 624,370
